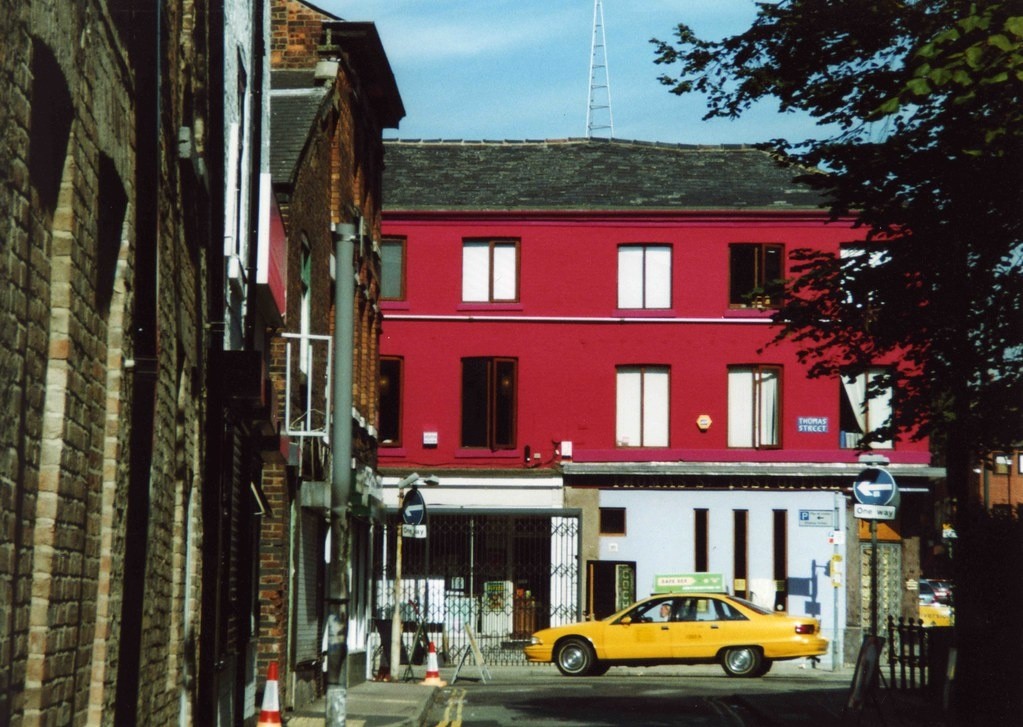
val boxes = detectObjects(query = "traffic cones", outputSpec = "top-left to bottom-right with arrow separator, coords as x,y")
254,659 -> 284,727
419,641 -> 448,689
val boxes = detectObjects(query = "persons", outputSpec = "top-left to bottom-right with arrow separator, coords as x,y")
921,542 -> 952,579
641,603 -> 672,623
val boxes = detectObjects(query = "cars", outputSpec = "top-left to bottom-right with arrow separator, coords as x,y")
524,572 -> 829,678
918,579 -> 953,605
